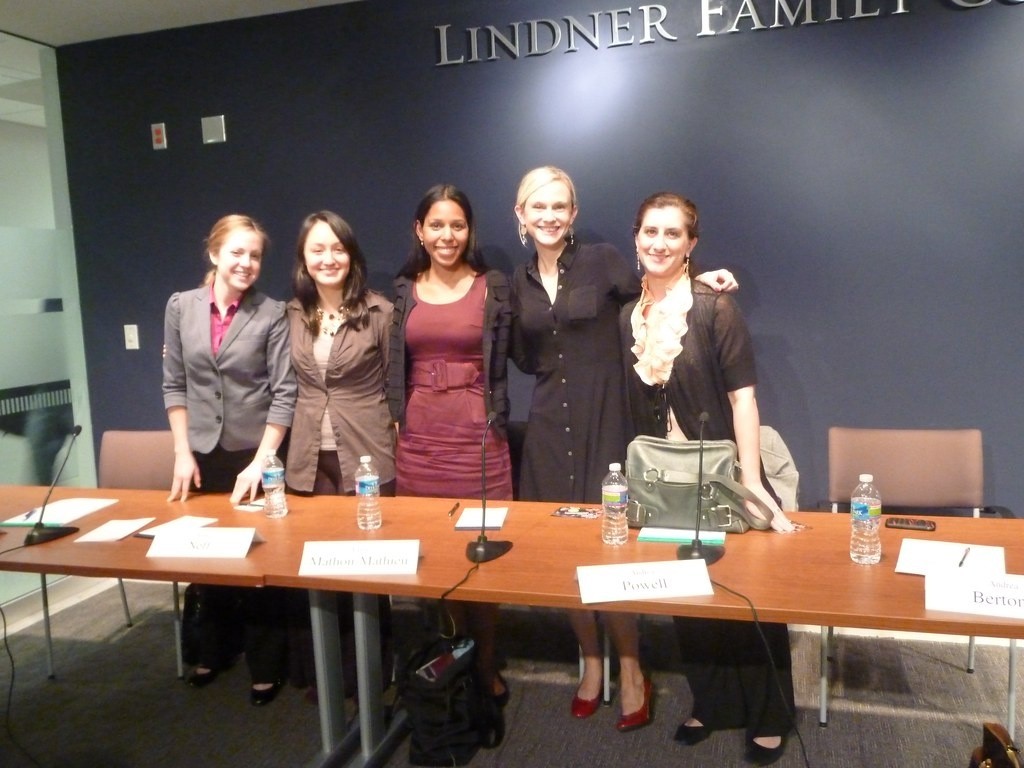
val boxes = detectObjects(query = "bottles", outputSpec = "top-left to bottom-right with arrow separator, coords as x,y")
600,462 -> 630,545
260,450 -> 289,519
354,455 -> 382,530
849,473 -> 882,565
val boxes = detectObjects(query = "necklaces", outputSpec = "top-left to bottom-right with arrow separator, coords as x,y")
316,303 -> 349,335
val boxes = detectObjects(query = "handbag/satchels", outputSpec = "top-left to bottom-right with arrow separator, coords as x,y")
181,583 -> 233,665
623,435 -> 750,532
395,635 -> 506,768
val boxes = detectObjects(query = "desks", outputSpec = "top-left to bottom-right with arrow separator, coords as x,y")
1,483 -> 1024,768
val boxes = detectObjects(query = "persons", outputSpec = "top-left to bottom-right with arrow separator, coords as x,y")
620,189 -> 794,767
160,214 -> 295,703
377,181 -> 515,704
506,165 -> 741,732
284,210 -> 398,678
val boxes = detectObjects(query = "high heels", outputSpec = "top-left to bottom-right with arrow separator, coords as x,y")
571,673 -> 603,719
618,678 -> 654,731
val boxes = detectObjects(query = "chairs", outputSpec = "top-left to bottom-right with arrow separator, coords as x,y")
758,425 -> 799,511
820,426 -> 1017,746
41,431 -> 187,678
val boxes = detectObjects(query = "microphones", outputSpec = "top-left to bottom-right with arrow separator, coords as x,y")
465,411 -> 514,563
23,426 -> 82,545
676,412 -> 726,566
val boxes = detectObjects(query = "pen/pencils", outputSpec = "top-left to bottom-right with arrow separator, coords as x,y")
239,502 -> 264,507
23,507 -> 36,521
958,548 -> 970,567
448,501 -> 461,516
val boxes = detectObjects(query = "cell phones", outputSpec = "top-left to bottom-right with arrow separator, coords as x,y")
885,518 -> 936,531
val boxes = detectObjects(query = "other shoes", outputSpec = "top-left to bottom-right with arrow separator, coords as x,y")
478,668 -> 509,705
747,729 -> 790,763
673,719 -> 713,747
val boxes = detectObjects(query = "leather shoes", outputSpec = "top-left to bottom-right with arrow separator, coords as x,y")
249,680 -> 284,706
189,667 -> 219,687
